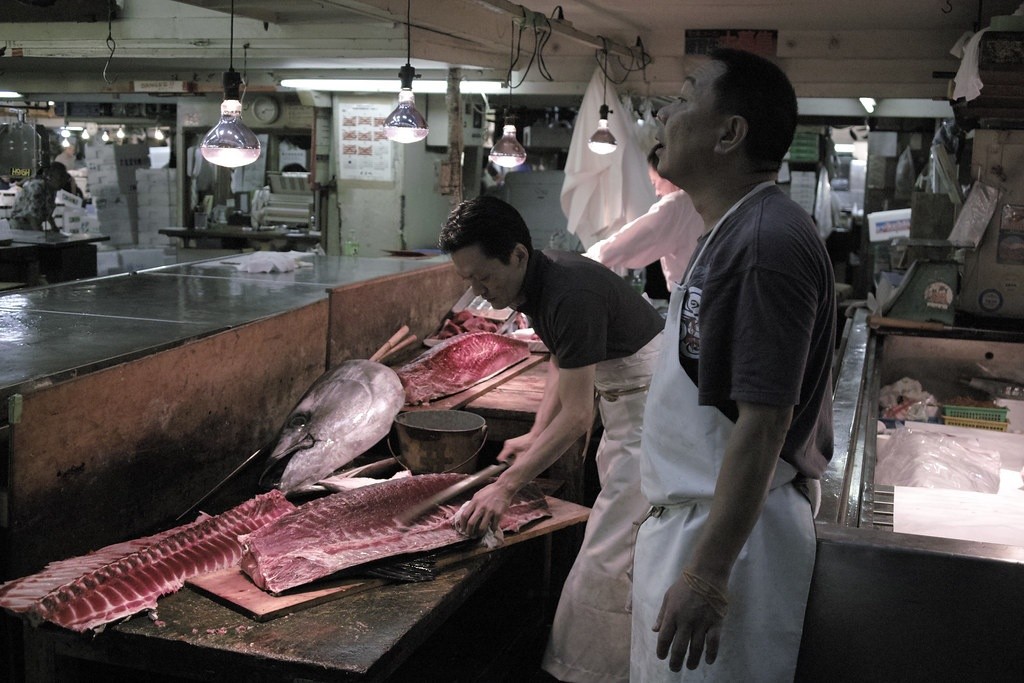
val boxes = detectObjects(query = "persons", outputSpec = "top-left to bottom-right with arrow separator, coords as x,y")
436,194 -> 666,683
627,48 -> 839,683
580,143 -> 707,298
10,162 -> 87,288
52,145 -> 87,169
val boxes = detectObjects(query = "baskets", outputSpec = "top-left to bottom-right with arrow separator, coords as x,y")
941,404 -> 1009,432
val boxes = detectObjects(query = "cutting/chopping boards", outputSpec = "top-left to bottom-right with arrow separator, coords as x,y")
390,348 -> 550,410
186,494 -> 592,621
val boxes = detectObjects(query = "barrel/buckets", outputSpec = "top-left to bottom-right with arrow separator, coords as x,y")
387,409 -> 489,476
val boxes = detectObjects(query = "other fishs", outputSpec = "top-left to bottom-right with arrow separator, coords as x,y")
0,470 -> 550,634
261,330 -> 528,491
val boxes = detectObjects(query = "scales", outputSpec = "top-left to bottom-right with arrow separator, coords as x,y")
881,237 -> 975,325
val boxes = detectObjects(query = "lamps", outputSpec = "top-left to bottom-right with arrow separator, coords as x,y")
201,0 -> 262,168
588,38 -> 620,158
384,0 -> 430,143
488,16 -> 527,169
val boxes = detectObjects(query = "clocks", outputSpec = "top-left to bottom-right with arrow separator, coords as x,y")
254,98 -> 277,124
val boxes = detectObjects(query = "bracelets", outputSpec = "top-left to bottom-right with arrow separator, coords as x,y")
681,568 -> 728,618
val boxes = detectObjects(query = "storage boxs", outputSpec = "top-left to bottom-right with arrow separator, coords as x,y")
0,141 -> 177,247
866,208 -> 913,243
